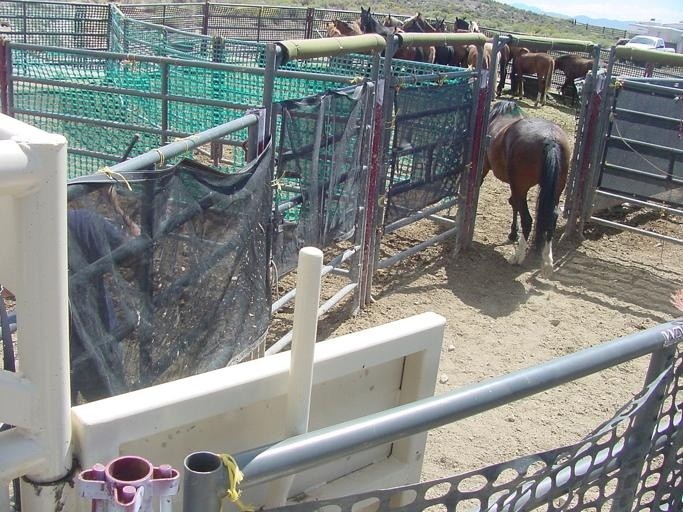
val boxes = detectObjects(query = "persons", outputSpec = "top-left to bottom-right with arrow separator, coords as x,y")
66,177 -> 144,407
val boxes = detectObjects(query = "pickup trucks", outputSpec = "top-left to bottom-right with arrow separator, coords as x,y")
625,35 -> 675,54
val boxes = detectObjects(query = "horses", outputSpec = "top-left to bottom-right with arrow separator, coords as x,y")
553,51 -> 606,98
325,5 -> 512,100
481,100 -> 572,269
513,46 -> 555,107
